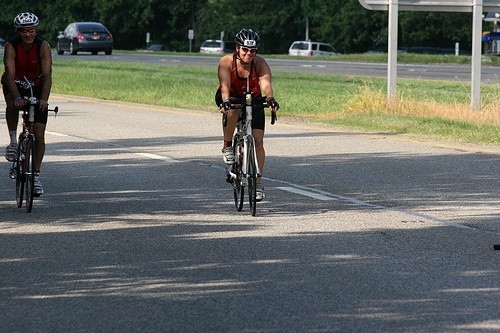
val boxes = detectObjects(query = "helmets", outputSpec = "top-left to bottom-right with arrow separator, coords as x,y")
235,29 -> 260,47
13,12 -> 40,28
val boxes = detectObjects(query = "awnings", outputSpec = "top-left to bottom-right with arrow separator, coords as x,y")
482,32 -> 500,41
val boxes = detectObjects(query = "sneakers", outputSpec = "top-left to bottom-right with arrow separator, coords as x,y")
6,144 -> 18,160
33,179 -> 44,195
256,185 -> 265,200
222,146 -> 235,164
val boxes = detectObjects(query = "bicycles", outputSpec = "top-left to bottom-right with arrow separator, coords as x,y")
8,96 -> 58,213
218,91 -> 276,216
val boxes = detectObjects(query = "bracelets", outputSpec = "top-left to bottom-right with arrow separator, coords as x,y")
16,97 -> 21,100
224,98 -> 229,102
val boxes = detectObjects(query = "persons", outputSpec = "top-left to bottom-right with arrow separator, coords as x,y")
1,12 -> 52,195
215,28 -> 279,202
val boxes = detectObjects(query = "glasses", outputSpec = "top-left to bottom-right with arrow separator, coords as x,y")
19,28 -> 35,33
239,46 -> 257,53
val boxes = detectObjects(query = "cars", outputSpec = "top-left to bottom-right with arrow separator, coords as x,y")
147,43 -> 180,52
199,39 -> 237,55
56,22 -> 114,55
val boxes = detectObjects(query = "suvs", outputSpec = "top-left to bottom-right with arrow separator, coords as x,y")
288,41 -> 343,56
